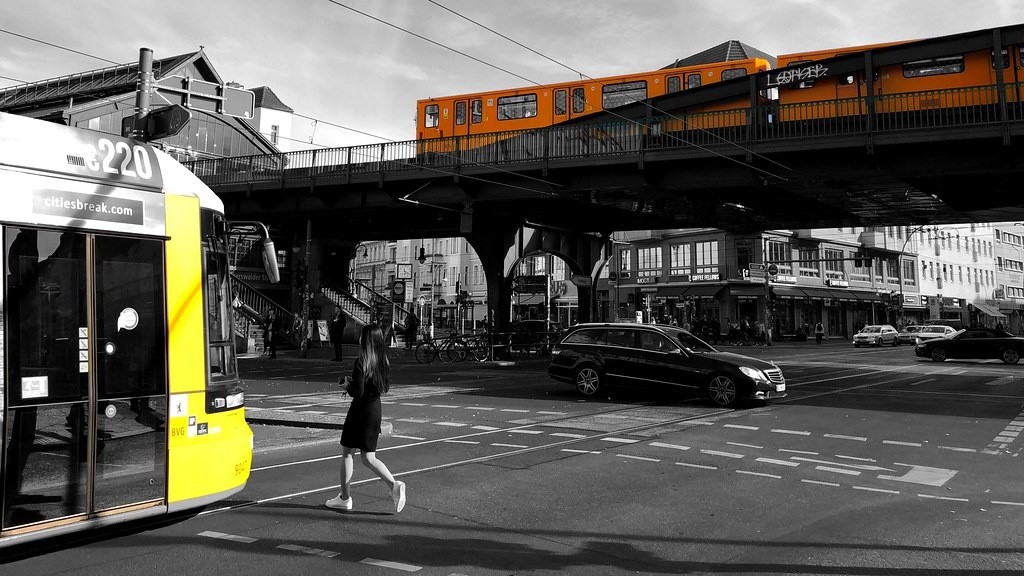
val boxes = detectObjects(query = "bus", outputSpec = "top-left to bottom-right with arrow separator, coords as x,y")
0,111 -> 280,554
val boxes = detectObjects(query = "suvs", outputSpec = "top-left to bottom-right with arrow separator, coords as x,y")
548,321 -> 787,408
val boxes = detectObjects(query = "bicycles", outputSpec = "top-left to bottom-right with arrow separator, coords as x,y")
414,324 -> 494,365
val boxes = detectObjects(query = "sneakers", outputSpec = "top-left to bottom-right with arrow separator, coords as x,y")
326,492 -> 352,509
389,481 -> 406,513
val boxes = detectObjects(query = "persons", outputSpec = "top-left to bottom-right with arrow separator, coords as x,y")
325,324 -> 406,515
329,305 -> 346,362
231,285 -> 243,327
656,312 -> 825,347
291,312 -> 302,352
261,308 -> 278,359
403,307 -> 420,350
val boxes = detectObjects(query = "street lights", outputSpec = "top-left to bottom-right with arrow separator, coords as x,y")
348,243 -> 368,294
591,244 -> 634,321
418,238 -> 436,340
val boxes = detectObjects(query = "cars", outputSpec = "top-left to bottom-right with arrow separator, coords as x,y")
852,325 -> 898,348
512,319 -> 566,334
915,325 -> 957,345
914,328 -> 1024,364
897,325 -> 920,345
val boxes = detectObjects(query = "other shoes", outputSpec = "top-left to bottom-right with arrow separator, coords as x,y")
331,356 -> 343,362
